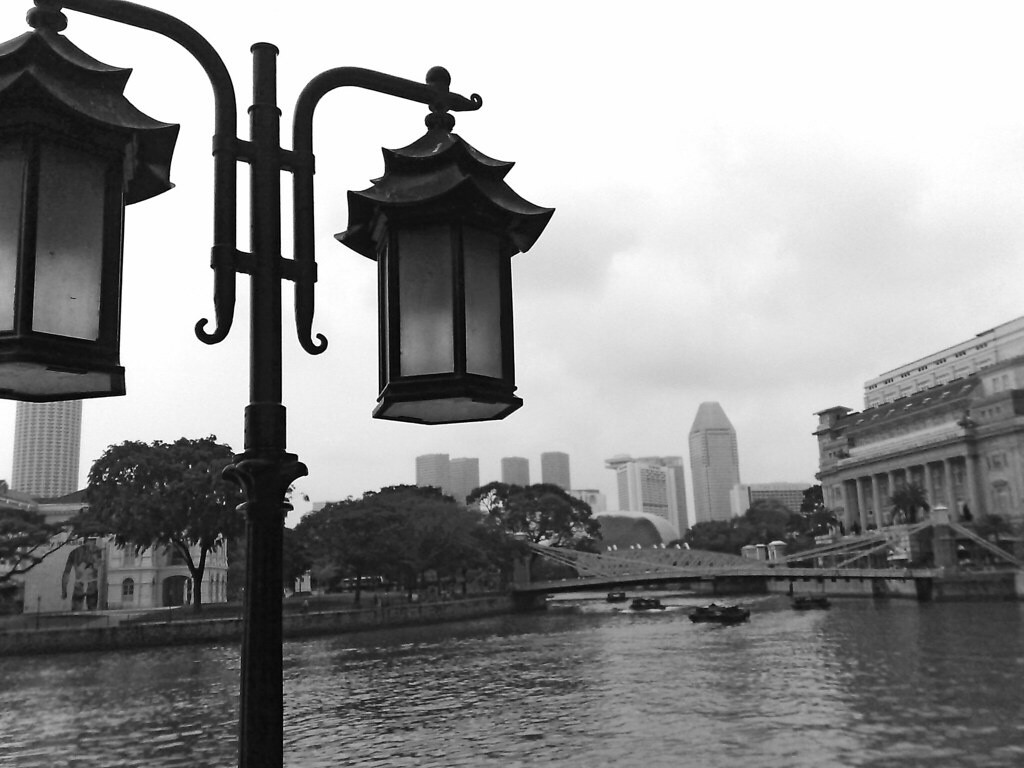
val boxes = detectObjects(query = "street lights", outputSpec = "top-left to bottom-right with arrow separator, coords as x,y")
1,0 -> 560,767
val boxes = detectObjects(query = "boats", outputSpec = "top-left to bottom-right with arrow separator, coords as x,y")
790,595 -> 834,611
630,596 -> 668,610
687,604 -> 751,623
605,591 -> 628,602
